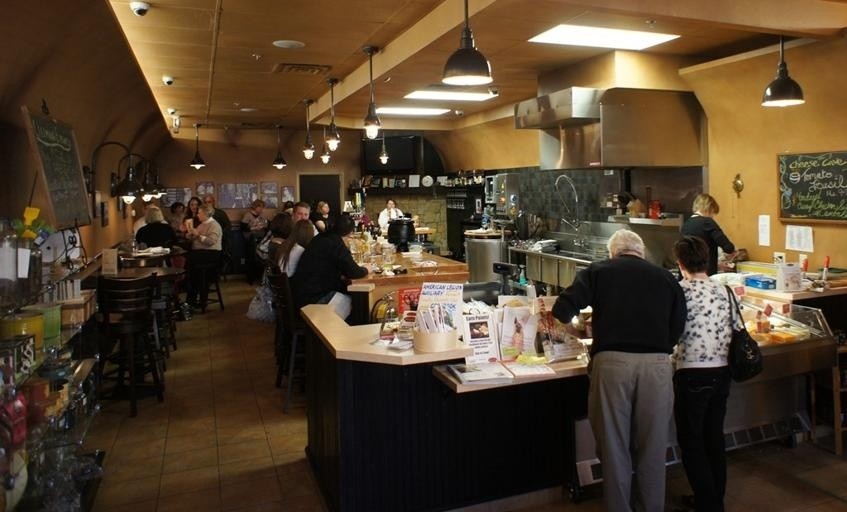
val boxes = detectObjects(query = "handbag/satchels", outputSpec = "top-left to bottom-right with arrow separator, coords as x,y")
246,270 -> 270,323
725,285 -> 764,386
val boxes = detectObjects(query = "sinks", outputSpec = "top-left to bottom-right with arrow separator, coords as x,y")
560,252 -> 582,257
576,255 -> 604,262
542,249 -> 569,255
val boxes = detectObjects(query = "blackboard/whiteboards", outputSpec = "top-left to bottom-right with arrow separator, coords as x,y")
20,105 -> 91,231
775,149 -> 847,226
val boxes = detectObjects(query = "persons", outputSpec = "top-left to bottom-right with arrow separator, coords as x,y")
680,193 -> 736,275
204,195 -> 232,233
135,204 -> 180,250
291,201 -> 321,236
378,199 -> 405,238
508,317 -> 525,358
279,218 -> 315,278
292,213 -> 373,320
310,200 -> 331,231
185,204 -> 224,309
166,202 -> 186,231
133,200 -> 169,237
239,198 -> 265,240
551,229 -> 688,512
183,197 -> 201,229
268,213 -> 294,275
677,236 -> 740,512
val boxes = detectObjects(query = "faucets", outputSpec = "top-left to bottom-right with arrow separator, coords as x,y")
554,175 -> 581,246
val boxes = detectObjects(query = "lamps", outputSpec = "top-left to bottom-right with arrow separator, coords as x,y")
319,125 -> 331,165
82,140 -> 145,207
379,130 -> 389,165
272,124 -> 287,170
362,45 -> 381,140
325,77 -> 342,152
760,35 -> 806,109
135,158 -> 159,203
189,122 -> 206,170
110,151 -> 146,207
402,89 -> 500,102
442,0 -> 494,85
527,21 -> 682,52
153,174 -> 167,200
301,98 -> 316,161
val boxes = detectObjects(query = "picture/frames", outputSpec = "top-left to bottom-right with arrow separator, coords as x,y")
260,180 -> 279,196
92,190 -> 103,219
217,182 -> 260,210
280,185 -> 296,204
100,201 -> 109,229
124,204 -> 131,220
261,194 -> 280,211
158,186 -> 193,209
117,195 -> 123,213
195,180 -> 216,201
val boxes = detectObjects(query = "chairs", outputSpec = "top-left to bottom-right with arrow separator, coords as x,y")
266,270 -> 316,415
262,258 -> 283,275
90,270 -> 166,418
192,237 -> 226,313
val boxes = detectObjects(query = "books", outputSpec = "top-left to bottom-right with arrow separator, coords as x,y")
446,362 -> 515,385
460,312 -> 499,364
536,311 -> 585,363
500,305 -> 538,361
502,357 -> 557,383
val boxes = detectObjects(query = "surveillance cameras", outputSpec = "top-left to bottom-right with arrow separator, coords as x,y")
488,86 -> 501,98
162,75 -> 174,86
130,1 -> 150,18
454,108 -> 466,118
168,109 -> 175,114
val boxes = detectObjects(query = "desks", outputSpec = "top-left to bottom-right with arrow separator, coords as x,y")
118,244 -> 189,268
81,265 -> 189,298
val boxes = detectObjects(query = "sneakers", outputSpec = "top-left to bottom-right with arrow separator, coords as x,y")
679,494 -> 695,508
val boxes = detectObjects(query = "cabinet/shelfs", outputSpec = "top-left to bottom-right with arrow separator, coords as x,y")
1,257 -> 99,512
